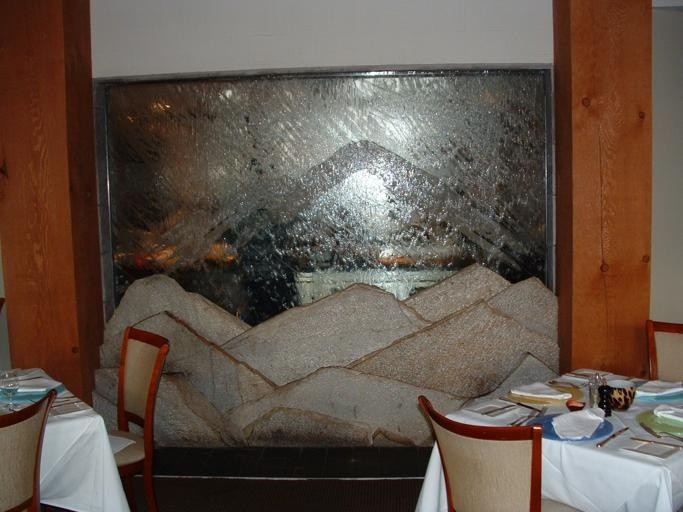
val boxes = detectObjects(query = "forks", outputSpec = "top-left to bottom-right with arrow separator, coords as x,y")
508,406 -> 548,427
640,423 -> 683,441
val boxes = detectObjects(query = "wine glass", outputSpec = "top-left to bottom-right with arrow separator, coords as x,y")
0,371 -> 20,412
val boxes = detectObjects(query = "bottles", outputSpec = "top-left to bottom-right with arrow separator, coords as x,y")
589,370 -> 611,417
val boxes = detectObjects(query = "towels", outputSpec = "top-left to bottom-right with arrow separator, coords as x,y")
511,380 -> 682,440
0,377 -> 62,392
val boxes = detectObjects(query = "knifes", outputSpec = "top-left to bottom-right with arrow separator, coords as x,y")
630,437 -> 683,451
596,427 -> 630,447
481,405 -> 516,416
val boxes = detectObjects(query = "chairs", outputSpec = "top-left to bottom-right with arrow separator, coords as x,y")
106,326 -> 174,511
646,318 -> 683,380
0,389 -> 58,511
417,393 -> 545,508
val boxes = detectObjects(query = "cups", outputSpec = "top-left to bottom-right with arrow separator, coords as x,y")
607,378 -> 635,412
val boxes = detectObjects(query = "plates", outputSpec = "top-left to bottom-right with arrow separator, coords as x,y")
528,413 -> 613,442
507,382 -> 583,406
634,408 -> 683,436
632,381 -> 683,403
0,378 -> 66,404
